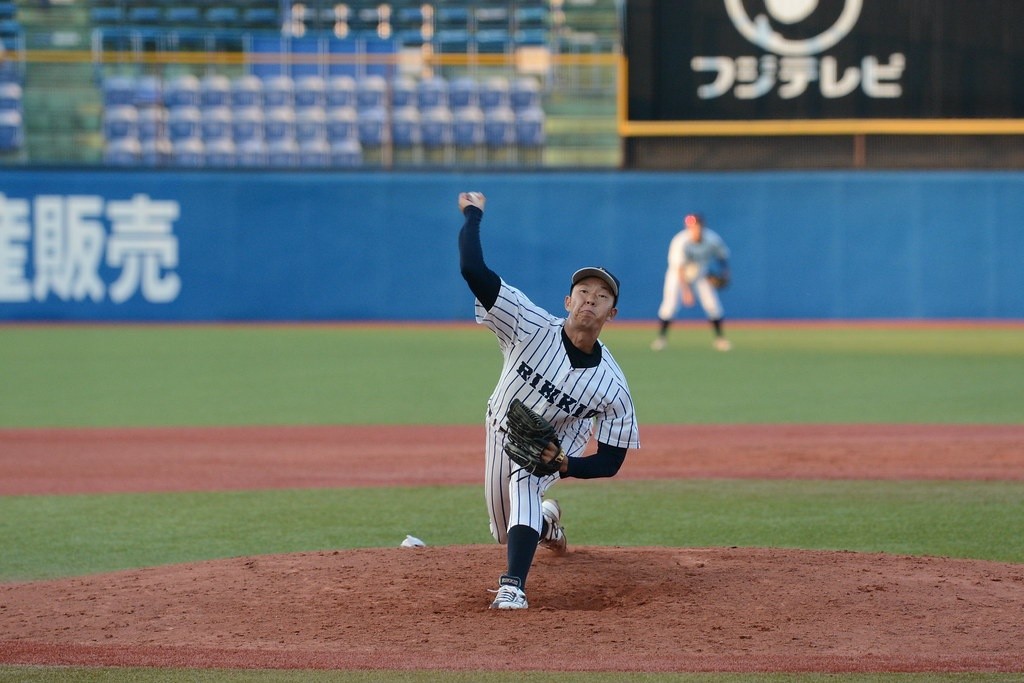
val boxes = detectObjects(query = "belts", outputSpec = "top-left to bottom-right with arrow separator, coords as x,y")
488,404 -> 508,433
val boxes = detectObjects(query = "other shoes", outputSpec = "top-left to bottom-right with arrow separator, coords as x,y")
712,339 -> 732,351
652,336 -> 668,350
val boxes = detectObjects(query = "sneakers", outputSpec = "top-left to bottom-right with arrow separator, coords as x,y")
537,499 -> 567,553
486,573 -> 528,610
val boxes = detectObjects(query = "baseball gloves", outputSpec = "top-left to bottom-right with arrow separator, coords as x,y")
706,272 -> 727,288
501,397 -> 567,491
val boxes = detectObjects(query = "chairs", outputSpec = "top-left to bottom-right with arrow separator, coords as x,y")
0,0 -> 550,172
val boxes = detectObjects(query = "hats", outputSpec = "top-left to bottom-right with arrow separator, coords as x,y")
572,266 -> 620,297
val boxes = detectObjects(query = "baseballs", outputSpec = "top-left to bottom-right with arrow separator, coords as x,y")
468,191 -> 479,203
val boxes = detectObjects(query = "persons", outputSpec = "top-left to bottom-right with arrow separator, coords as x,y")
459,191 -> 641,609
649,213 -> 733,351
